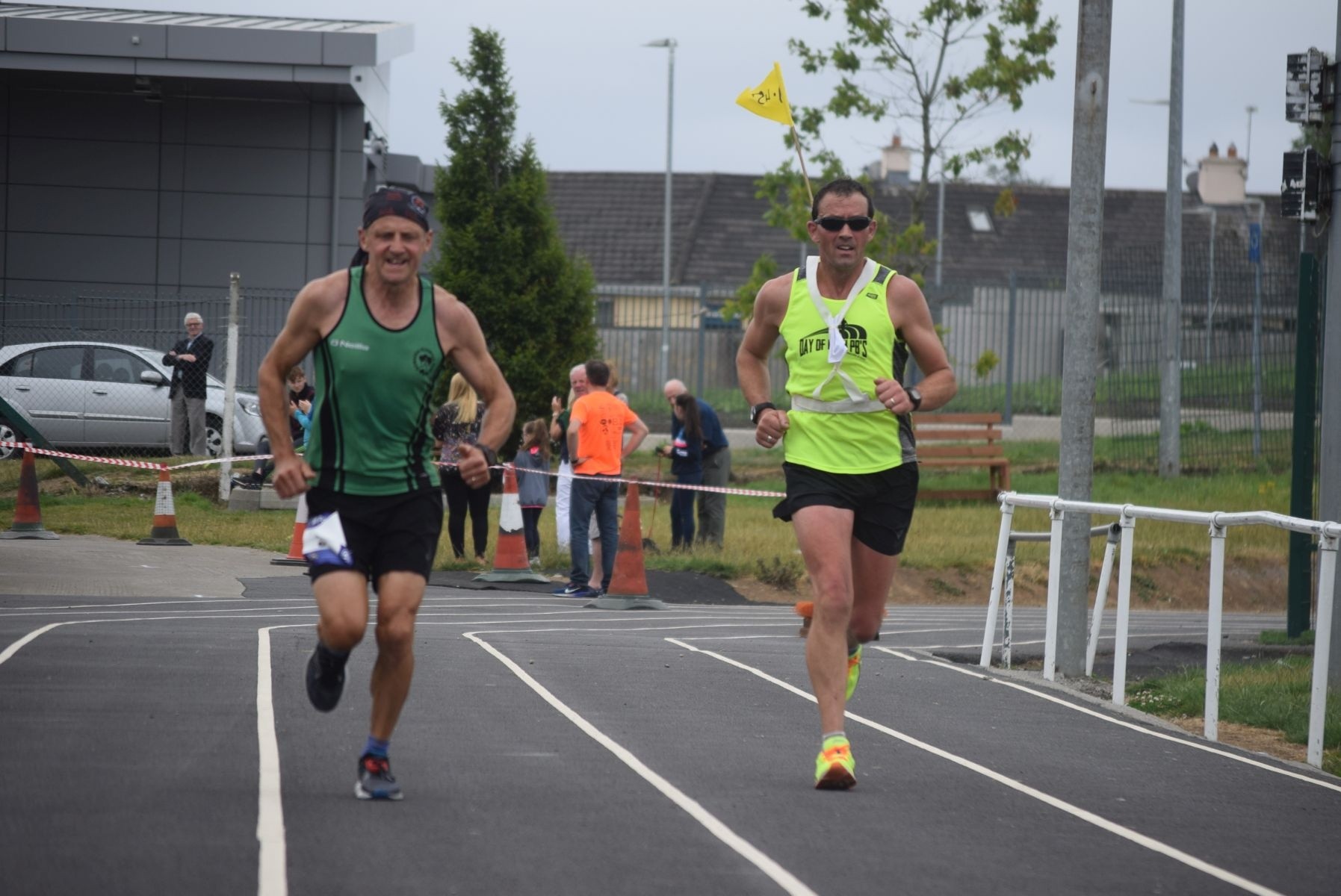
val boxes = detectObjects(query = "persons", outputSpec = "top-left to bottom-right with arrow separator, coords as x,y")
432,371 -> 494,566
663,378 -> 731,561
511,417 -> 551,570
256,179 -> 519,804
161,311 -> 214,458
570,363 -> 604,591
550,356 -> 651,599
232,363 -> 316,491
658,394 -> 704,559
733,175 -> 958,793
550,388 -> 594,584
287,396 -> 317,452
603,358 -> 629,409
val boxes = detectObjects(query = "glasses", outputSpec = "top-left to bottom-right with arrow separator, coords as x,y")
814,215 -> 872,232
186,322 -> 202,326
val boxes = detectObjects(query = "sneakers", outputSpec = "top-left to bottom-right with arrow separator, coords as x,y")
232,476 -> 263,490
355,753 -> 405,800
814,736 -> 857,791
551,582 -> 595,597
305,639 -> 350,713
845,644 -> 862,702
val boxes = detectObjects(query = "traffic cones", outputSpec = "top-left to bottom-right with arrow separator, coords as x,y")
470,461 -> 550,585
136,462 -> 193,546
0,437 -> 61,540
581,473 -> 670,611
269,492 -> 309,567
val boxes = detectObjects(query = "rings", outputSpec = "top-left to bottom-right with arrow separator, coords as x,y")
767,436 -> 772,441
471,475 -> 476,483
890,398 -> 896,405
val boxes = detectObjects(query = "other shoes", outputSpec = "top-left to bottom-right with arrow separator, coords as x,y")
455,555 -> 540,567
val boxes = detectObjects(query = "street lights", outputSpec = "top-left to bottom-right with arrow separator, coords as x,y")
1235,197 -> 1264,455
642,38 -> 679,390
1181,205 -> 1216,369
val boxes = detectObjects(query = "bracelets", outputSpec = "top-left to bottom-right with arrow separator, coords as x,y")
552,413 -> 560,417
571,458 -> 580,464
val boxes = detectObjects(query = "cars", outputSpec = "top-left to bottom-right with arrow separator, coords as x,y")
0,340 -> 269,461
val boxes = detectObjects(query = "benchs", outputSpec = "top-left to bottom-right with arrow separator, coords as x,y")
909,411 -> 1012,503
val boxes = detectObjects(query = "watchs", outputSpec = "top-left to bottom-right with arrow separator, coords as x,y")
904,385 -> 924,413
750,402 -> 775,425
473,442 -> 498,468
